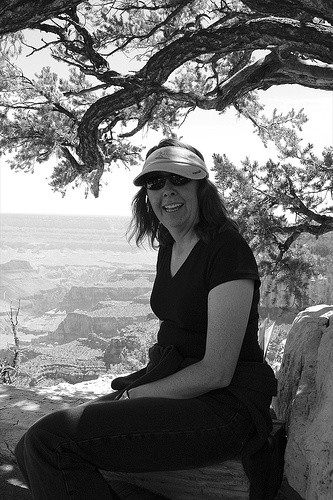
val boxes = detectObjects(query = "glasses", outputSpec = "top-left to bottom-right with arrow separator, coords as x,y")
141,172 -> 192,190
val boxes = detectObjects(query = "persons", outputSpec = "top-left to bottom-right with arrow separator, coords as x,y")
15,139 -> 279,500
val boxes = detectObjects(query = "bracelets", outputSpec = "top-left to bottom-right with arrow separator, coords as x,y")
122,389 -> 130,400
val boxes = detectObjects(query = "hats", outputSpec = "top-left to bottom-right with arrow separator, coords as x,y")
133,146 -> 209,185
